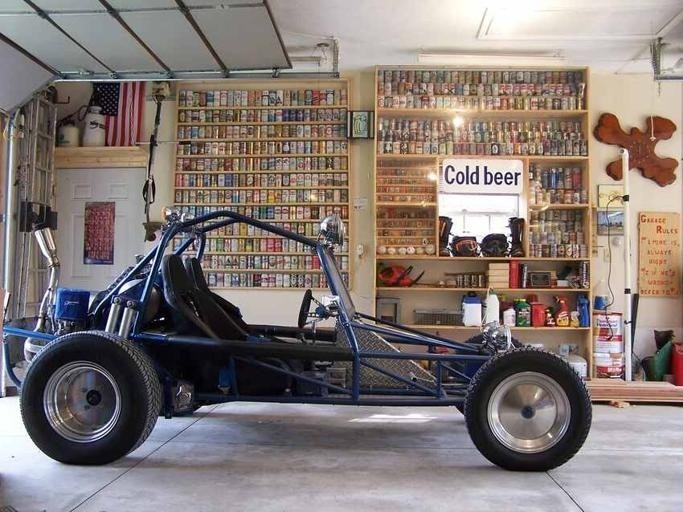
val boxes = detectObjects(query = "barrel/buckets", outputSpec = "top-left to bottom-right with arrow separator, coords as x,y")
592,309 -> 624,378
82,106 -> 106,146
58,121 -> 79,147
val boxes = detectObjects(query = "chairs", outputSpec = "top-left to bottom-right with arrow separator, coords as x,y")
160,253 -> 296,396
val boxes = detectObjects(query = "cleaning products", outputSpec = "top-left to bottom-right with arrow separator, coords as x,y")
462,293 -> 590,327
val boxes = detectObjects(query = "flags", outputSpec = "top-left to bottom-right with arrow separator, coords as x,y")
90,81 -> 146,148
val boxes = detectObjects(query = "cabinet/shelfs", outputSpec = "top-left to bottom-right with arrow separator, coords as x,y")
367,65 -> 595,387
169,80 -> 353,290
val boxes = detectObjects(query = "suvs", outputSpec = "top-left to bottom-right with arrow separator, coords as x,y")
2,201 -> 593,472
